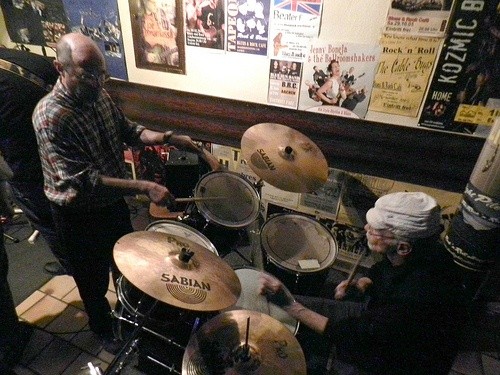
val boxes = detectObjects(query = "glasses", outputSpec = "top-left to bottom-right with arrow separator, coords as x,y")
61,64 -> 111,87
367,227 -> 396,240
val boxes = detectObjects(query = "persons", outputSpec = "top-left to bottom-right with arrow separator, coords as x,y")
306,60 -> 369,109
0,32 -> 203,355
235,17 -> 268,35
272,60 -> 300,77
258,192 -> 458,375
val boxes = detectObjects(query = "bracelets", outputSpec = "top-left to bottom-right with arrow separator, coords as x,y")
163,131 -> 174,143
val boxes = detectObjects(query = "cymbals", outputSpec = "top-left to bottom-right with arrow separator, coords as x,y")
241,122 -> 328,193
112,231 -> 242,311
182,308 -> 307,375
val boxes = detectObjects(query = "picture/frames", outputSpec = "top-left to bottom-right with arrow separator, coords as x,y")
129,0 -> 185,74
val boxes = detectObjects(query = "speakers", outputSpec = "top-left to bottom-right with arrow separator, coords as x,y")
164,149 -> 201,212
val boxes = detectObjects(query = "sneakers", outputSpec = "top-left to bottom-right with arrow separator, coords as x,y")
90,322 -> 124,355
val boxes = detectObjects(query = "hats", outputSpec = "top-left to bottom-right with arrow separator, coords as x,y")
366,193 -> 441,238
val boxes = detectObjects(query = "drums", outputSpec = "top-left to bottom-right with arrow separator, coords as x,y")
255,214 -> 339,297
183,169 -> 260,255
218,266 -> 299,338
117,219 -> 220,324
